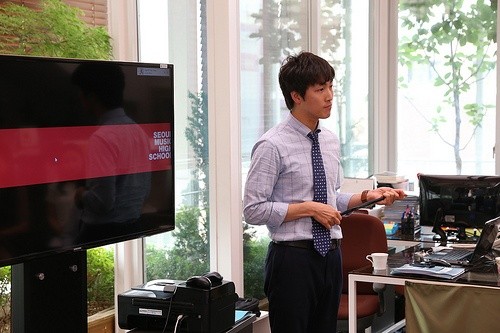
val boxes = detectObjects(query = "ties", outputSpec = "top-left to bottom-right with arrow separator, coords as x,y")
307,131 -> 332,257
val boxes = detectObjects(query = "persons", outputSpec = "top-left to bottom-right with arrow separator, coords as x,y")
69,62 -> 151,242
243,52 -> 407,333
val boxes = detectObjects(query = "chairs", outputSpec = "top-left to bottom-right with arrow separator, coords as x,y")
336,212 -> 389,333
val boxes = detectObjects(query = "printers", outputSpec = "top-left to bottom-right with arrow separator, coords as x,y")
116,279 -> 238,333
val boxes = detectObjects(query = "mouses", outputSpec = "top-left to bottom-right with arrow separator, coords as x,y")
186,275 -> 212,289
429,259 -> 450,267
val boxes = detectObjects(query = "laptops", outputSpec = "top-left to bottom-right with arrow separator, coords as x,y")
423,217 -> 500,266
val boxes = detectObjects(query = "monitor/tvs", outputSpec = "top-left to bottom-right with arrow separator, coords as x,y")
417,173 -> 500,243
0,53 -> 175,268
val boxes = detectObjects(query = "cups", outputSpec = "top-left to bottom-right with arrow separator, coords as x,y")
401,217 -> 414,235
420,225 -> 433,234
366,253 -> 389,270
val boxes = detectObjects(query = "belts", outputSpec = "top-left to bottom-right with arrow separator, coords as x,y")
275,238 -> 342,250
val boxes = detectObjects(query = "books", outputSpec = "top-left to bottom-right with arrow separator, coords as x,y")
381,195 -> 421,234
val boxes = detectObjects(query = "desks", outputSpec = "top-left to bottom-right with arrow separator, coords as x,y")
348,241 -> 500,333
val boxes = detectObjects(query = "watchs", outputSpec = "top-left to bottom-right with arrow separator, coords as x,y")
361,190 -> 375,210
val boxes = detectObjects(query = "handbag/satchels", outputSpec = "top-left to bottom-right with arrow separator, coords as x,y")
235,298 -> 261,318
